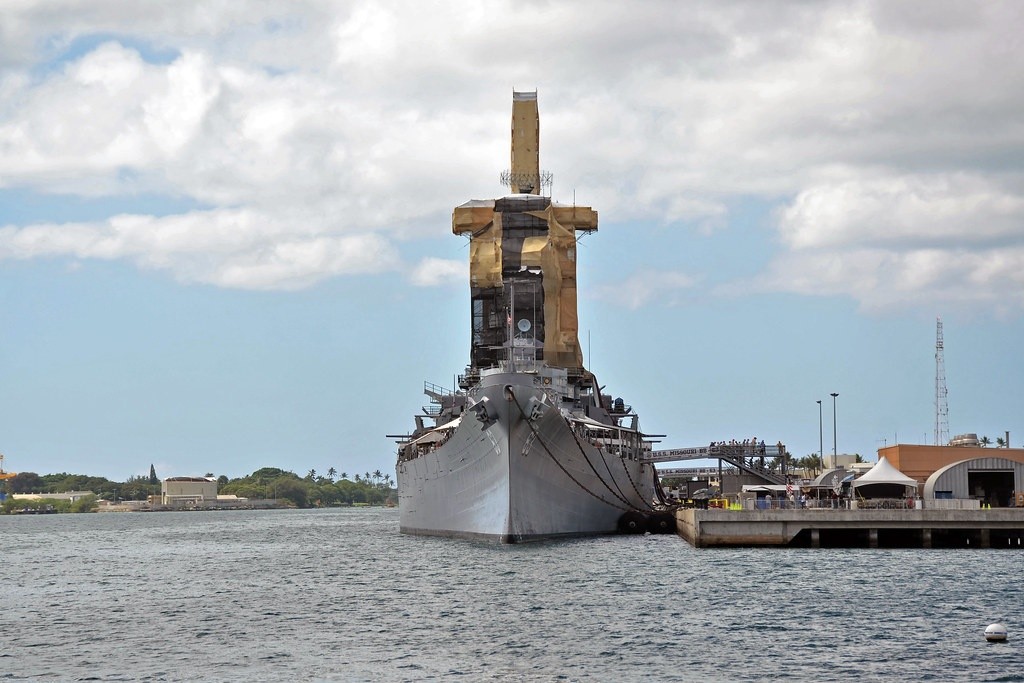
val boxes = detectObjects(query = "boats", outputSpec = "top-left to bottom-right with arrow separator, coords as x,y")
393,87 -> 672,546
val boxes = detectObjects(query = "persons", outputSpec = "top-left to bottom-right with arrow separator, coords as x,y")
800,492 -> 805,509
777,441 -> 784,455
765,494 -> 772,508
789,493 -> 796,504
710,436 -> 766,454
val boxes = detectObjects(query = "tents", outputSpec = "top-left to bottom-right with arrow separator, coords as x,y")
851,454 -> 918,488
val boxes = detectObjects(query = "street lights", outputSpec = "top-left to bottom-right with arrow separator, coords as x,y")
830,392 -> 840,470
817,400 -> 823,474
